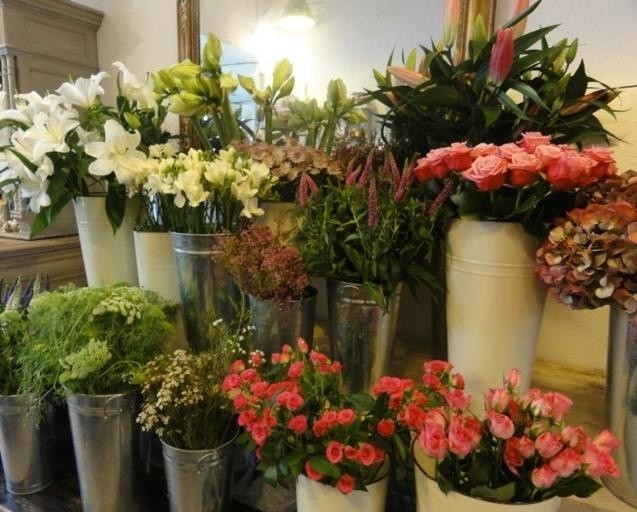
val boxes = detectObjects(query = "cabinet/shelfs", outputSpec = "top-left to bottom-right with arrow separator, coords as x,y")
0,0 -> 106,302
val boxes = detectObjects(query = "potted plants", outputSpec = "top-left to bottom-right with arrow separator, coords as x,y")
1,302 -> 61,504
36,289 -> 165,510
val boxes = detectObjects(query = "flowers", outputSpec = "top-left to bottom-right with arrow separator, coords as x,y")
237,57 -> 372,155
534,166 -> 636,314
211,228 -> 308,311
398,361 -> 620,508
365,1 -> 624,208
126,140 -> 271,236
221,338 -> 473,494
295,146 -> 454,314
149,30 -> 243,150
136,294 -> 260,453
0,58 -> 163,241
229,139 -> 342,201
412,130 -> 618,241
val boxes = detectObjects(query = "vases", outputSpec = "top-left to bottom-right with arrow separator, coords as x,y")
445,218 -> 549,414
327,274 -> 402,396
410,431 -> 560,512
168,228 -> 224,336
72,191 -> 138,291
248,287 -> 318,359
159,426 -> 240,512
601,304 -> 637,510
252,202 -> 303,253
133,230 -> 181,310
294,454 -> 392,512
